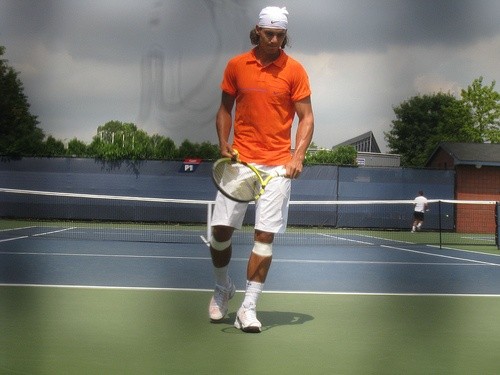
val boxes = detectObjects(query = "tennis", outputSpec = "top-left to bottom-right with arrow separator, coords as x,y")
445,214 -> 449,218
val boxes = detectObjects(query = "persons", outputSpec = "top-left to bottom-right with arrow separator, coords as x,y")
410,191 -> 428,233
207,5 -> 315,330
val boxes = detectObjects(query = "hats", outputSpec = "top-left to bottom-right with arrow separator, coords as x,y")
257,6 -> 289,30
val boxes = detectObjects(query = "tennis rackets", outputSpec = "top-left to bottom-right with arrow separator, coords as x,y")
212,149 -> 265,202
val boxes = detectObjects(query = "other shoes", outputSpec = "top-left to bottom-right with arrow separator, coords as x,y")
416,225 -> 421,232
410,231 -> 415,233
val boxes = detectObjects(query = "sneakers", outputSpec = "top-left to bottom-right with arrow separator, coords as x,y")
208,279 -> 235,320
234,303 -> 262,332
